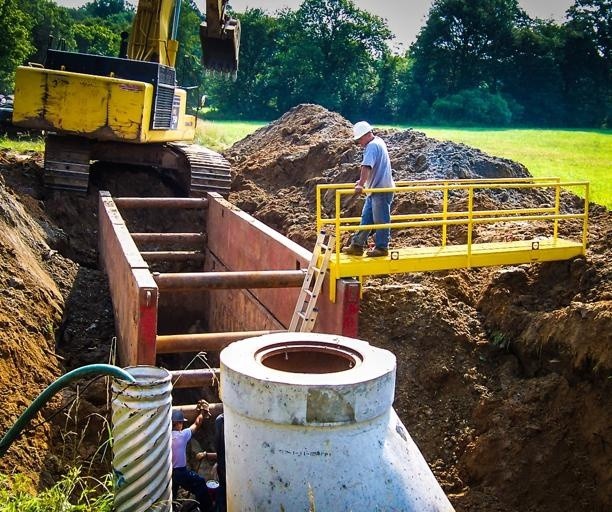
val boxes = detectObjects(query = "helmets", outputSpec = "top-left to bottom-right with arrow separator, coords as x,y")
351,119 -> 374,142
171,411 -> 189,421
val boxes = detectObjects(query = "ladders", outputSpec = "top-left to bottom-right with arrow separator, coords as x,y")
288,230 -> 336,332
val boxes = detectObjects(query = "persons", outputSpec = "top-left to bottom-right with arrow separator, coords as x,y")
342,121 -> 395,257
172,399 -> 226,512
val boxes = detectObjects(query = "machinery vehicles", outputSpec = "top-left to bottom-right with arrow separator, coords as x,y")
12,0 -> 241,201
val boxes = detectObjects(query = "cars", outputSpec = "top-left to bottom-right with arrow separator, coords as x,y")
0,94 -> 13,122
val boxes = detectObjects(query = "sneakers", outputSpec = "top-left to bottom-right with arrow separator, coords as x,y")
342,243 -> 364,256
367,247 -> 389,258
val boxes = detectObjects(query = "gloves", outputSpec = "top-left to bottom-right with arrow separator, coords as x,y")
198,399 -> 211,413
